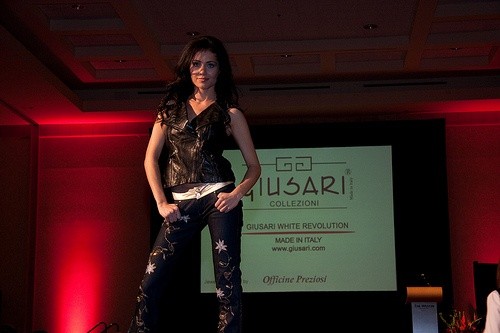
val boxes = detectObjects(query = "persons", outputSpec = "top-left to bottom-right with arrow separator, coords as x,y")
126,36 -> 262,332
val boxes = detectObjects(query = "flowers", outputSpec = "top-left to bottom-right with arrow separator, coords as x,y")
439,306 -> 483,333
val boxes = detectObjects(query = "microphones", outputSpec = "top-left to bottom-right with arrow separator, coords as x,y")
421,274 -> 426,280
